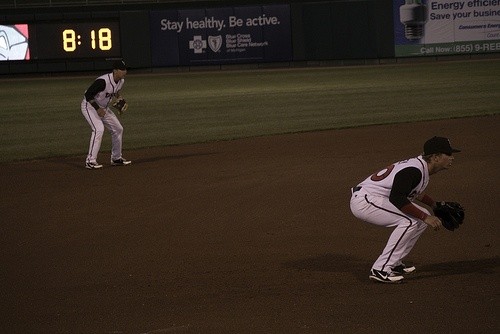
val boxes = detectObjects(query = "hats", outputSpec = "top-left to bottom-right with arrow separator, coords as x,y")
424,137 -> 462,155
113,60 -> 130,69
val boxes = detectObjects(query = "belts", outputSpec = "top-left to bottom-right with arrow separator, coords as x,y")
353,187 -> 362,193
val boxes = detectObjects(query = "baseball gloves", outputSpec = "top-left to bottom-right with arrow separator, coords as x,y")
432,201 -> 465,231
111,97 -> 128,112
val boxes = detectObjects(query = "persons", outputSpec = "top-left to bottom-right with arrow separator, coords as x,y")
81,60 -> 131,169
349,136 -> 454,282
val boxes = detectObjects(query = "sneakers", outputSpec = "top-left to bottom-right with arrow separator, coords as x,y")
368,268 -> 405,283
85,162 -> 104,169
391,263 -> 416,275
111,158 -> 132,166
